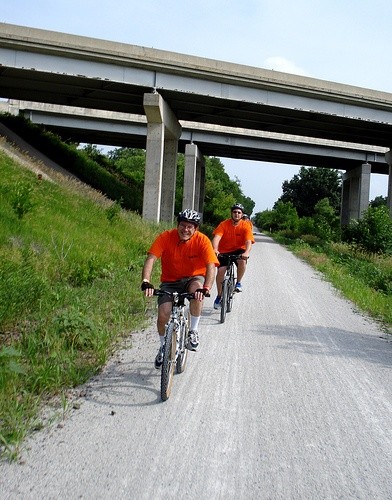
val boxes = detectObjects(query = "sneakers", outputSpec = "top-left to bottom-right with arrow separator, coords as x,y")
235,282 -> 242,291
188,329 -> 200,351
154,347 -> 162,369
214,296 -> 221,309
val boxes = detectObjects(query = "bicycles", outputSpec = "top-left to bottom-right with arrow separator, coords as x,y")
218,254 -> 249,323
153,288 -> 211,401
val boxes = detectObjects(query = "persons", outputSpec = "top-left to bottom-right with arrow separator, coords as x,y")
242,214 -> 250,221
142,209 -> 216,370
212,202 -> 252,310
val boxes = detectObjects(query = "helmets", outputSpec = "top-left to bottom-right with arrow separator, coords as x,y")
178,208 -> 200,225
243,214 -> 249,218
231,203 -> 244,212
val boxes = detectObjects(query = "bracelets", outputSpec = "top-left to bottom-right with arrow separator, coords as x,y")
203,286 -> 210,290
142,278 -> 150,283
